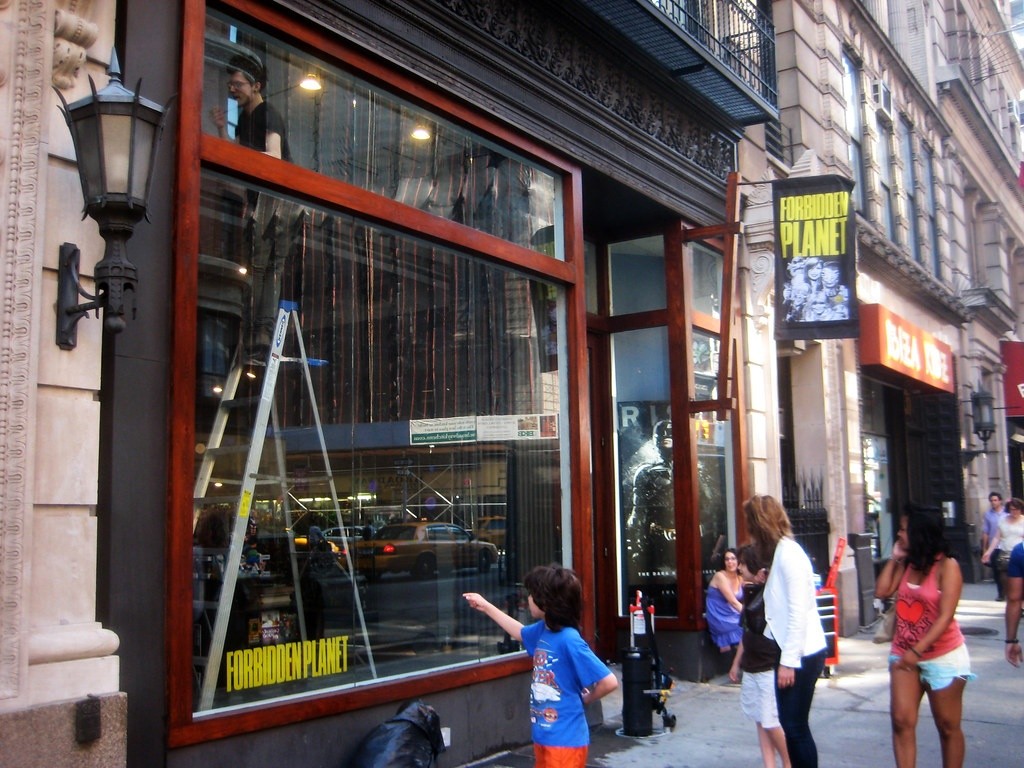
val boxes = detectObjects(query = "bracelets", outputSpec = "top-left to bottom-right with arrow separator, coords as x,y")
1004,639 -> 1018,643
908,645 -> 922,660
887,553 -> 904,564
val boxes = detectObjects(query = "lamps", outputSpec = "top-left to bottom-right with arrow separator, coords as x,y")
411,123 -> 430,140
299,73 -> 322,90
960,384 -> 1000,466
55,44 -> 178,352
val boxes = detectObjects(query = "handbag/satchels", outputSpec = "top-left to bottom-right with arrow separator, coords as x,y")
872,593 -> 897,643
744,583 -> 767,634
998,550 -> 1012,568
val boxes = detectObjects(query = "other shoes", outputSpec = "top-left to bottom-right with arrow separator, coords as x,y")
244,345 -> 268,366
995,596 -> 1005,601
726,677 -> 742,687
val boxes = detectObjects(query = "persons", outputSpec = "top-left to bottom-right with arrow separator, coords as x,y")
701,492 -> 829,768
240,534 -> 266,572
460,563 -> 619,768
623,417 -> 727,615
872,502 -> 971,768
978,491 -> 1024,670
302,525 -> 338,639
222,48 -> 301,358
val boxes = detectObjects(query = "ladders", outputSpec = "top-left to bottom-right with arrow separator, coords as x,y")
193,301 -> 379,714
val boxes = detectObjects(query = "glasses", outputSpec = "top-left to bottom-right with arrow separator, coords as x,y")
227,80 -> 250,88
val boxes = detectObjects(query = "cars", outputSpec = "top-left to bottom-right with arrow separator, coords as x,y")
293,526 -> 368,568
473,517 -> 506,549
339,522 -> 499,578
